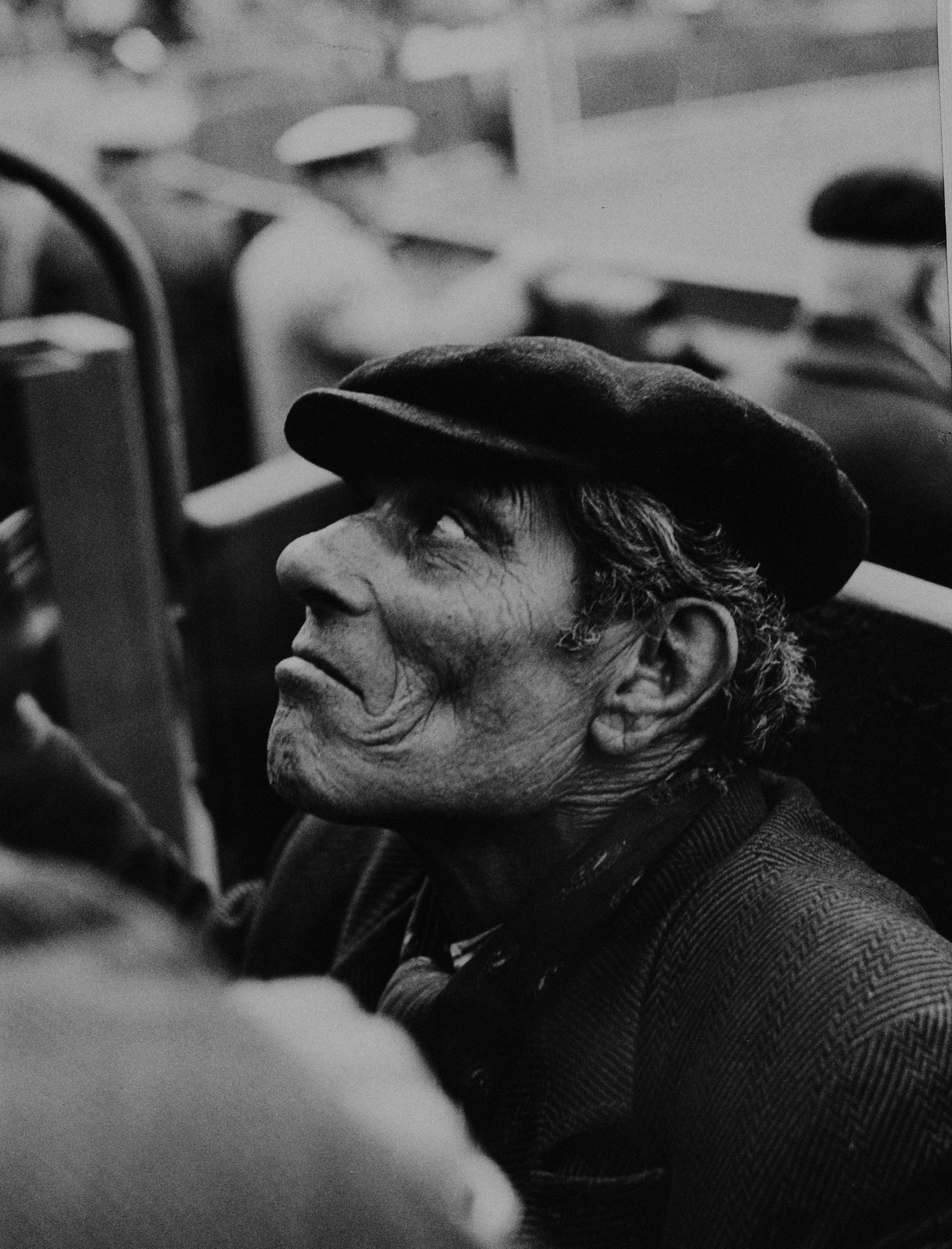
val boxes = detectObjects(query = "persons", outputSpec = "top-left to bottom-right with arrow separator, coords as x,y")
0,105 -> 952,1249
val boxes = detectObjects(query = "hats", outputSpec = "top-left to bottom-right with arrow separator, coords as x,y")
285,337 -> 870,607
811,171 -> 946,247
273,103 -> 416,163
